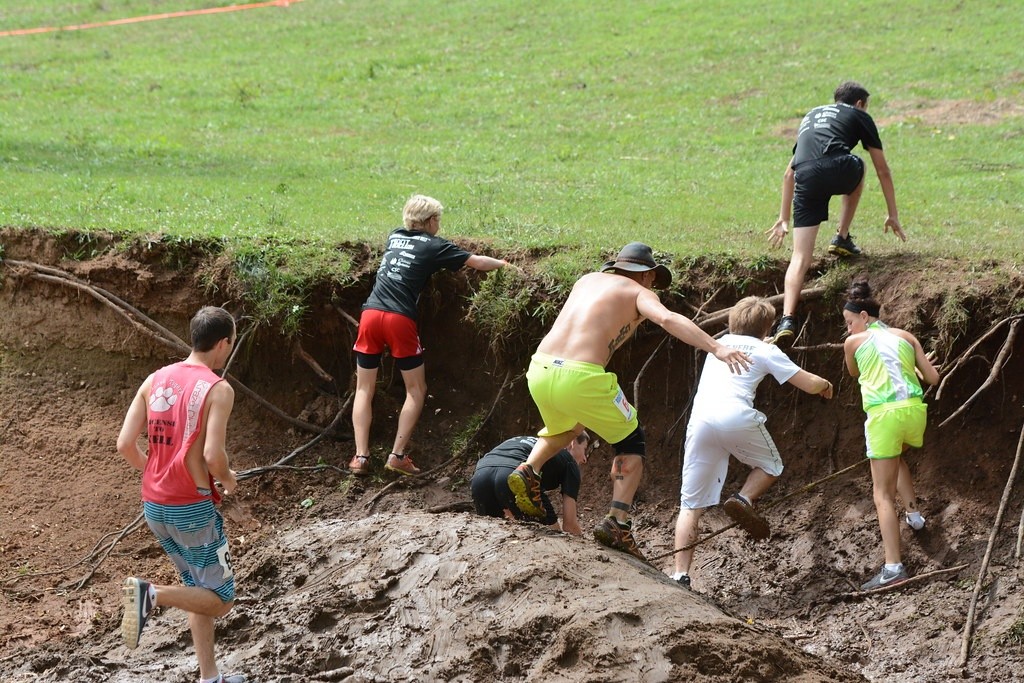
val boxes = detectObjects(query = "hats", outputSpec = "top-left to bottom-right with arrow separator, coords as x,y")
601,241 -> 672,289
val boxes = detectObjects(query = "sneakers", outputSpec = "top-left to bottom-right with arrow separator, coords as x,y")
593,512 -> 646,562
724,492 -> 770,534
673,577 -> 690,591
385,453 -> 420,477
348,454 -> 369,474
215,676 -> 244,683
828,232 -> 860,259
121,578 -> 153,649
770,319 -> 796,349
508,462 -> 547,516
860,564 -> 908,591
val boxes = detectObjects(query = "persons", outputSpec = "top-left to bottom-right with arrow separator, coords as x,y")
507,243 -> 753,566
349,196 -> 525,480
117,306 -> 246,682
668,296 -> 833,591
765,83 -> 907,345
472,430 -> 590,536
843,282 -> 939,590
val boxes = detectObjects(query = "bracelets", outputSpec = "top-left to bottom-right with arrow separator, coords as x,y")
824,383 -> 830,391
